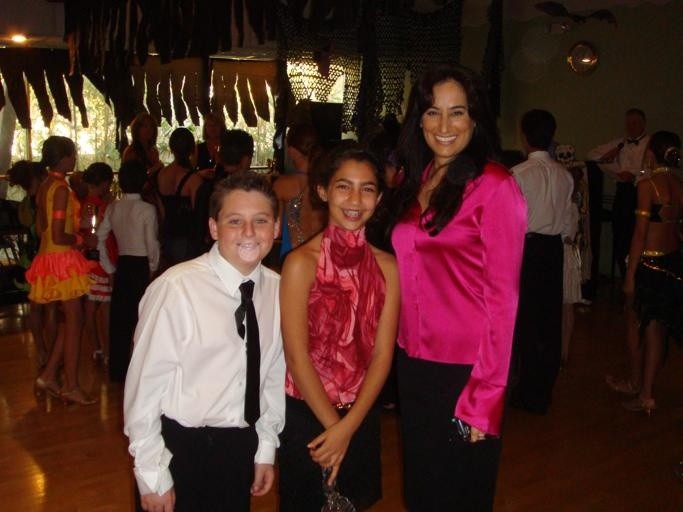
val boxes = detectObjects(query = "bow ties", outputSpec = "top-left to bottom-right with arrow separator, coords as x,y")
627,139 -> 638,145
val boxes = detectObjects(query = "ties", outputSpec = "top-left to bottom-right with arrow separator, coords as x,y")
234,280 -> 260,426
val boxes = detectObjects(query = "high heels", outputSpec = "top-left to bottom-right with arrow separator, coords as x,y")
620,398 -> 655,417
607,376 -> 641,396
60,387 -> 97,405
34,378 -> 61,397
